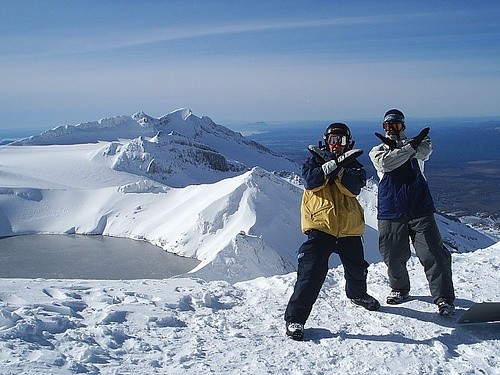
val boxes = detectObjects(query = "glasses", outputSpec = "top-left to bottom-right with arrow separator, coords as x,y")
383,124 -> 403,130
328,136 -> 349,146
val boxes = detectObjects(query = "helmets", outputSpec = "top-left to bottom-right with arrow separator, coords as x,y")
383,109 -> 406,123
324,123 -> 351,135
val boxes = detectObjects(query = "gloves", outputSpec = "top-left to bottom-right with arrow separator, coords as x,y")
322,149 -> 363,179
375,132 -> 397,148
410,127 -> 431,149
308,145 -> 335,162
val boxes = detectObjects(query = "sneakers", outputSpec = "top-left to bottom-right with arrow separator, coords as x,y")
438,301 -> 454,316
286,321 -> 304,341
351,293 -> 380,311
386,291 -> 411,304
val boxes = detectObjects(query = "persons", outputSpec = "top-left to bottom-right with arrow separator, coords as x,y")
284,123 -> 380,340
368,108 -> 456,316
